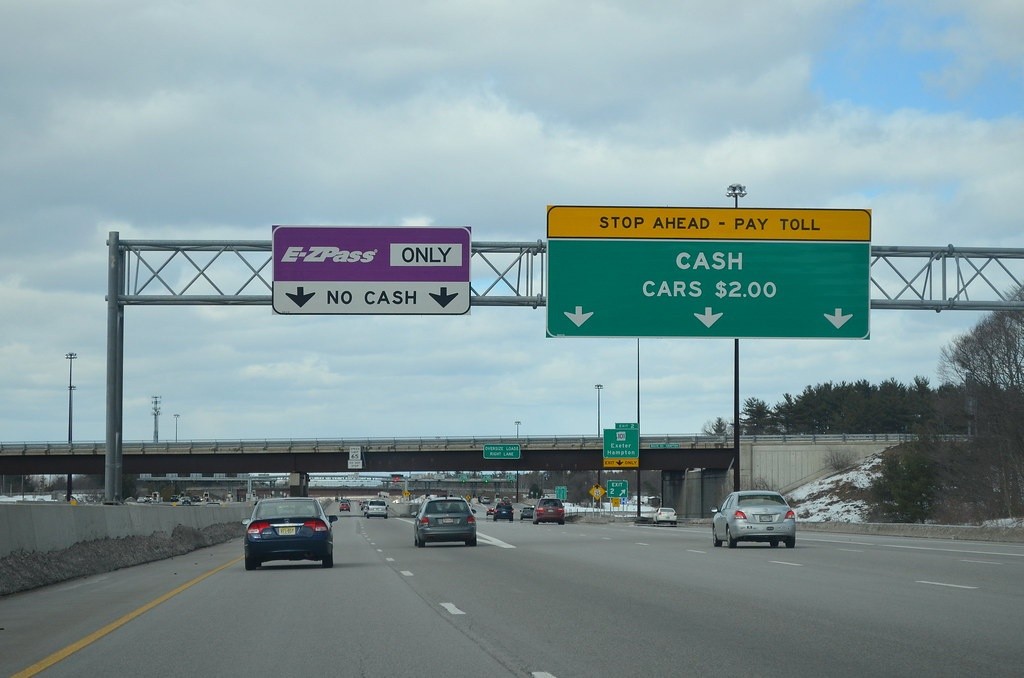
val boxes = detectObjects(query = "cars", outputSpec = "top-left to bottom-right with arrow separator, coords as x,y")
520,506 -> 535,520
710,491 -> 796,549
171,495 -> 178,502
137,497 -> 145,503
493,507 -> 514,523
181,497 -> 191,505
144,495 -> 153,502
339,498 -> 370,516
496,502 -> 512,509
503,497 -> 509,501
486,507 -> 494,516
653,508 -> 678,526
193,495 -> 201,502
505,499 -> 512,506
241,498 -> 339,570
533,498 -> 565,526
481,497 -> 490,504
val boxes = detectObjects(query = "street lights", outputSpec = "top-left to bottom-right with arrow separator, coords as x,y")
65,352 -> 77,501
595,384 -> 604,507
726,184 -> 747,491
515,420 -> 521,501
174,414 -> 180,444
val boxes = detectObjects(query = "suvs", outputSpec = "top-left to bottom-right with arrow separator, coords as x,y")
410,495 -> 477,548
366,499 -> 390,519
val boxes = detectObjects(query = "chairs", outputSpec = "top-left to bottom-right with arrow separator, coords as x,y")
295,507 -> 311,515
554,503 -> 558,506
265,507 -> 278,516
449,504 -> 460,512
544,502 -> 549,505
426,504 -> 437,512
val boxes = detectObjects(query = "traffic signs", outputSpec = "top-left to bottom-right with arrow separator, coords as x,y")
269,224 -> 472,315
603,428 -> 640,468
546,206 -> 872,342
